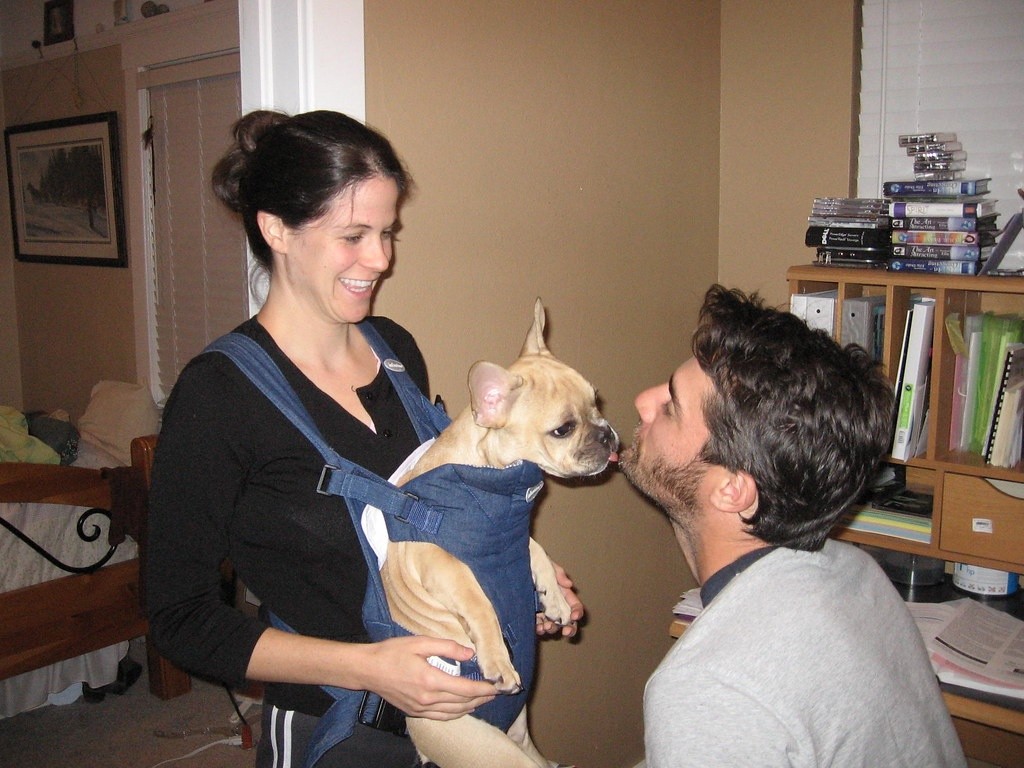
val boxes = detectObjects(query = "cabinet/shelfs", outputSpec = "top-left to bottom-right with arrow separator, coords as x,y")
670,265 -> 1024,768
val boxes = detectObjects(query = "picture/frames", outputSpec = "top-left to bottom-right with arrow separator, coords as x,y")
4,110 -> 130,269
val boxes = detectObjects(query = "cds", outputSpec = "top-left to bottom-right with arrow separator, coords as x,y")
883,552 -> 946,586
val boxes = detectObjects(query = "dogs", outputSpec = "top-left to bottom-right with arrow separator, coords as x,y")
378,295 -> 621,767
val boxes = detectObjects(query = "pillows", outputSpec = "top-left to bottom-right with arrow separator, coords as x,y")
74,379 -> 155,466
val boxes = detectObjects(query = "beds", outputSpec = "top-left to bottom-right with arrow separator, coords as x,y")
0,436 -> 190,722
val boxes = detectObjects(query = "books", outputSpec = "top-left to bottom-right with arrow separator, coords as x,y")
838,504 -> 932,544
906,602 -> 1024,698
888,297 -> 1024,467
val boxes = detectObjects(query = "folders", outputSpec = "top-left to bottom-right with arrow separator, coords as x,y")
891,301 -> 935,460
840,294 -> 885,368
792,289 -> 838,339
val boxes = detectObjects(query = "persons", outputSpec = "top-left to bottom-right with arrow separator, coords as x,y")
619,283 -> 967,768
145,111 -> 584,768
